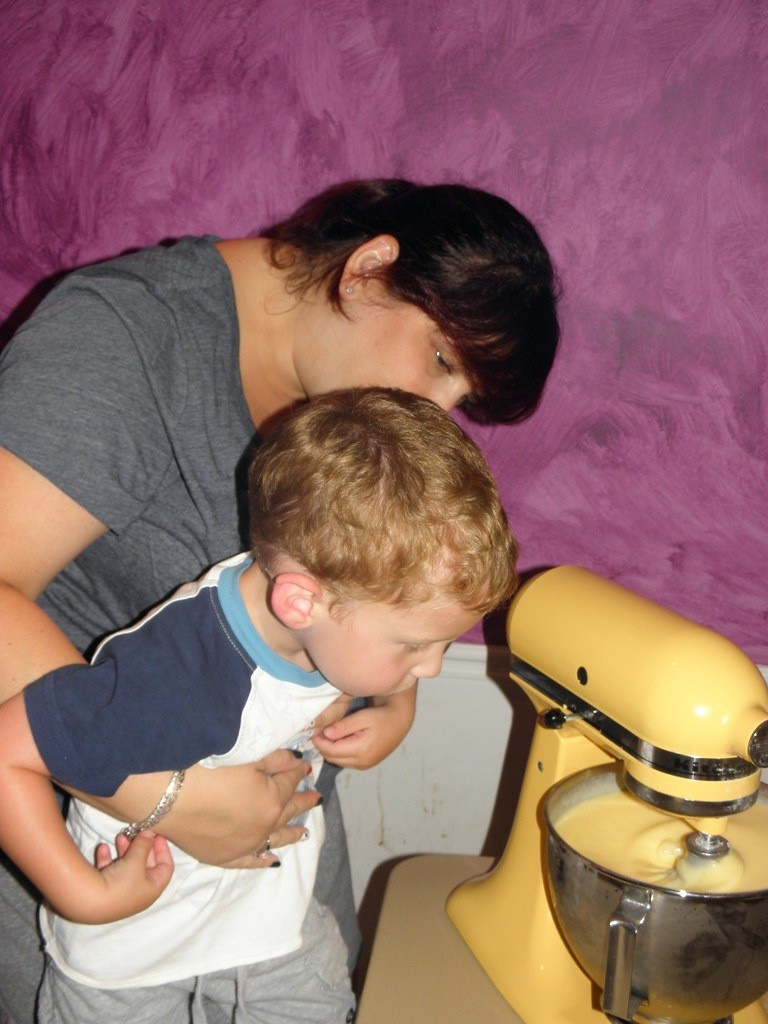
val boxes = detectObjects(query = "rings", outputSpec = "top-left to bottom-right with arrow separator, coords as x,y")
258,839 -> 271,859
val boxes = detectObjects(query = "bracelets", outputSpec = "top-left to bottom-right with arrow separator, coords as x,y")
124,769 -> 186,841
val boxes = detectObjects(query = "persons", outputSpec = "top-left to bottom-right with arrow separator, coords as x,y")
0,389 -> 520,1023
0,177 -> 564,1023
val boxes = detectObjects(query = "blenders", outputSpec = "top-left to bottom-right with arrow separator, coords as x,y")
445,563 -> 768,1024
